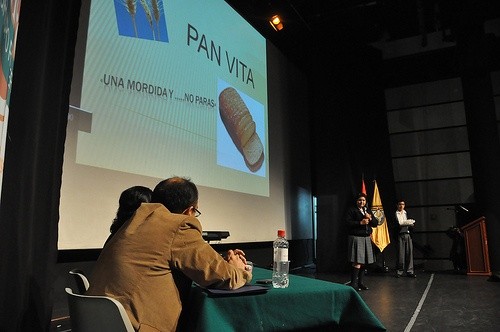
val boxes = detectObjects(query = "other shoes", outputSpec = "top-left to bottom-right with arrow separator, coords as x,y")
408,272 -> 415,278
394,275 -> 403,278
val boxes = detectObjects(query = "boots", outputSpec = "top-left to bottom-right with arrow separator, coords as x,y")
351,269 -> 362,292
359,269 -> 369,290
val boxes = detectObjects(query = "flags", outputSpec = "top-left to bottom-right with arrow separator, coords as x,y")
362,180 -> 367,196
369,184 -> 390,251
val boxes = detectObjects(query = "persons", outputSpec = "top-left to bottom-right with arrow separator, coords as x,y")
104,186 -> 153,247
394,201 -> 417,278
345,193 -> 379,291
86,177 -> 248,332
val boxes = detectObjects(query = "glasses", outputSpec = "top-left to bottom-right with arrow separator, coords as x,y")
183,206 -> 201,217
399,204 -> 405,205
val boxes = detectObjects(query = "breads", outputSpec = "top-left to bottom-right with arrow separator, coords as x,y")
219,86 -> 264,171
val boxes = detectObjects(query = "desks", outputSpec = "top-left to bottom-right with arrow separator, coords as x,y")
175,266 -> 388,332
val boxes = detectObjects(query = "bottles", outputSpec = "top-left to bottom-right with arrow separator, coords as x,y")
272,230 -> 290,289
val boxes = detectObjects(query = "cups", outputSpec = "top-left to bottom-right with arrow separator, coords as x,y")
246,260 -> 254,272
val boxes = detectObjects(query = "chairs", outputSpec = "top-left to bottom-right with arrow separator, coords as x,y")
69,268 -> 90,295
64,287 -> 136,332
